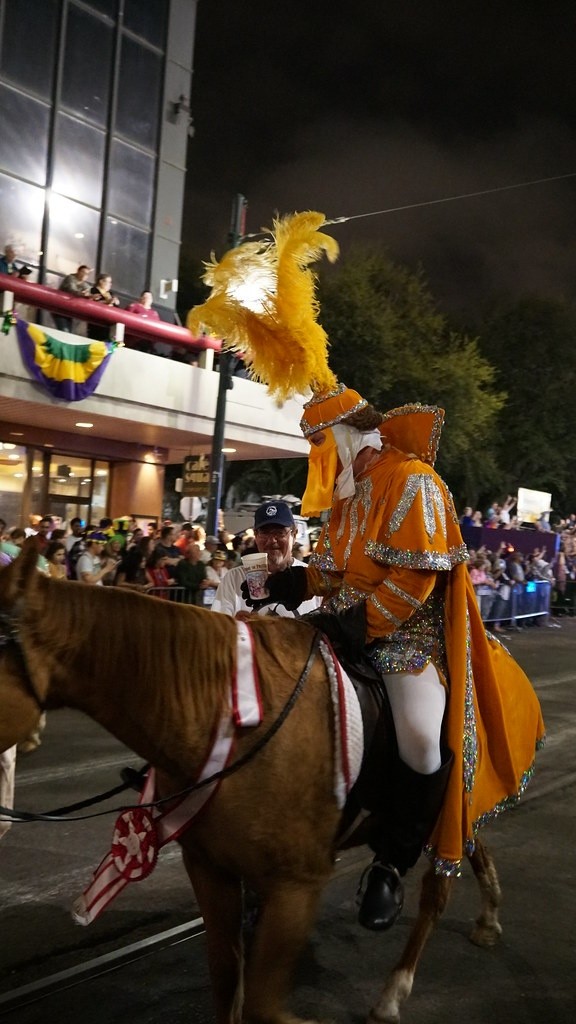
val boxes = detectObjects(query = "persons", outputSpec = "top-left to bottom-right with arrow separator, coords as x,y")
187,210 -> 546,934
0,495 -> 576,839
0,244 -> 29,317
123,290 -> 198,366
87,274 -> 120,343
50,266 -> 101,333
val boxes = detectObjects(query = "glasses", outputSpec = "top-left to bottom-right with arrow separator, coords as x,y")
257,529 -> 292,537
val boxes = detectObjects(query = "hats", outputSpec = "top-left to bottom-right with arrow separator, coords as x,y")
181,524 -> 193,530
85,532 -> 107,543
207,536 -> 221,544
255,502 -> 294,529
188,209 -> 368,437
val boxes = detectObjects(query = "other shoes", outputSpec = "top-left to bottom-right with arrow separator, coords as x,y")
493,624 -> 502,631
18,737 -> 37,753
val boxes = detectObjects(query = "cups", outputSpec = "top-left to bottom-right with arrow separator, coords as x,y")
241,552 -> 271,601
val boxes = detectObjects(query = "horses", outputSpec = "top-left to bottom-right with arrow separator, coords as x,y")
1,533 -> 547,1024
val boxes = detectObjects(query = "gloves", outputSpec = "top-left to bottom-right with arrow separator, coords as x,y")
241,566 -> 308,611
296,599 -> 367,647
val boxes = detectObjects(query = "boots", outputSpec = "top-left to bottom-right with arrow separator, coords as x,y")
358,744 -> 456,932
